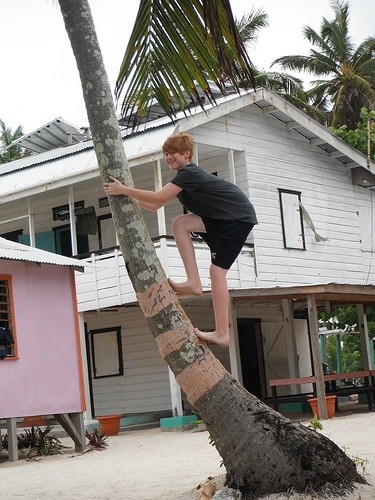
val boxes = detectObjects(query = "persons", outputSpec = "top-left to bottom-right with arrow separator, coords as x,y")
102,132 -> 259,348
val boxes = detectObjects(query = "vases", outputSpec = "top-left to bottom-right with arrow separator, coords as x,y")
307,395 -> 337,420
95,415 -> 122,436
